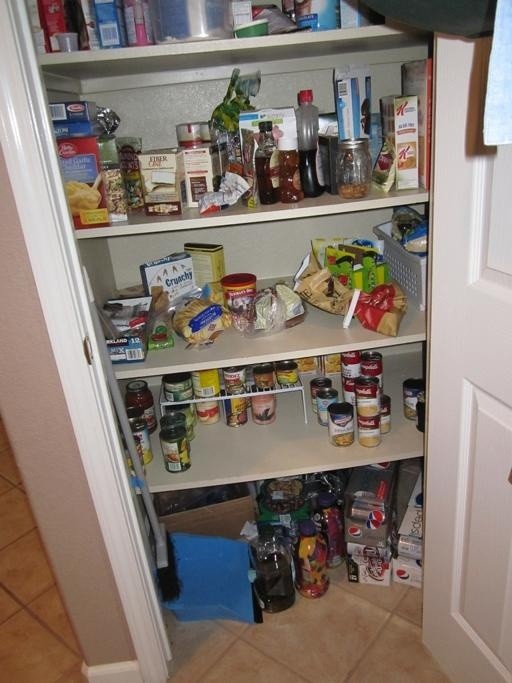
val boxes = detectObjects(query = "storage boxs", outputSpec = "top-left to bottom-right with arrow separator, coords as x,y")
158,494 -> 257,543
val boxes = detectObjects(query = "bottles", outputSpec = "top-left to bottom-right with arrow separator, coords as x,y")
336,139 -> 372,200
255,120 -> 280,204
313,491 -> 347,568
295,90 -> 326,198
290,519 -> 330,599
277,136 -> 305,203
247,526 -> 295,612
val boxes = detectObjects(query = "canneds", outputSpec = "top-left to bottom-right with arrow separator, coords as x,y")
355,376 -> 381,417
342,377 -> 356,406
164,373 -> 192,393
381,394 -> 391,435
402,378 -> 425,421
357,415 -> 382,447
316,388 -> 339,427
121,435 -> 144,471
276,361 -> 299,386
160,412 -> 187,432
163,389 -> 194,402
165,404 -> 194,441
253,365 -> 275,388
341,351 -> 361,379
327,402 -> 354,446
222,367 -> 246,394
127,417 -> 154,464
309,377 -> 332,413
195,401 -> 221,425
360,351 -> 383,393
159,426 -> 191,473
126,380 -> 157,434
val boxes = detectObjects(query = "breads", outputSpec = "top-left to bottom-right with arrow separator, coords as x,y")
173,299 -> 232,343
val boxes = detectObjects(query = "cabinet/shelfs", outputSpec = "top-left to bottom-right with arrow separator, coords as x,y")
35,24 -> 437,496
